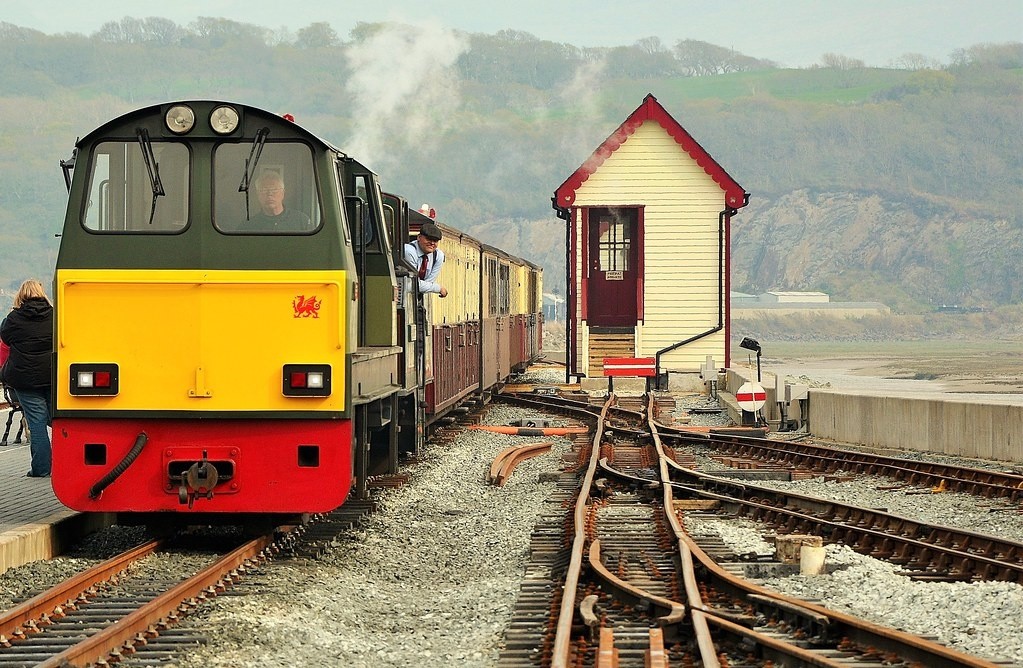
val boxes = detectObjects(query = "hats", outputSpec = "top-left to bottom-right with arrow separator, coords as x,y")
420,222 -> 442,242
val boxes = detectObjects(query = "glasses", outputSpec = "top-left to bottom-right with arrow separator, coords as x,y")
258,187 -> 283,194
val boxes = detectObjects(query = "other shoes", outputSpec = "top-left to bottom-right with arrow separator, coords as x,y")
27,471 -> 51,476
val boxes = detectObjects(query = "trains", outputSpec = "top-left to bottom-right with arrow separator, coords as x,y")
47,101 -> 544,534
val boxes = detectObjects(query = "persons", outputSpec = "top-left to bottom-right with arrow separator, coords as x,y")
0,280 -> 54,477
403,223 -> 448,298
237,172 -> 312,232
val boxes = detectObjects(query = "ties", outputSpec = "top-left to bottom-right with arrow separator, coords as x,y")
418,255 -> 428,280
